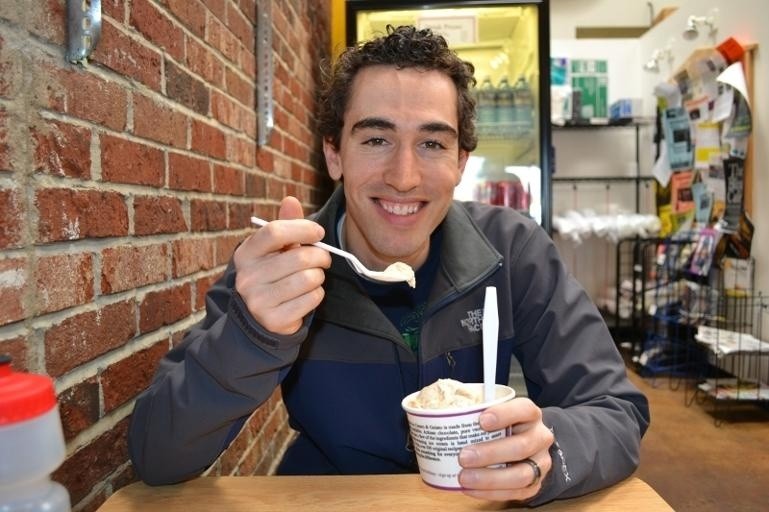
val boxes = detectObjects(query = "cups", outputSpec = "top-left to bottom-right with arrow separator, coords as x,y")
403,384 -> 518,489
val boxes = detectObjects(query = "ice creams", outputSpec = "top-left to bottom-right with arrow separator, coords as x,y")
383,261 -> 416,288
407,379 -> 485,411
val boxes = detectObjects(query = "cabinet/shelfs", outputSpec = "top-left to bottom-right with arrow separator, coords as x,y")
552,120 -> 659,215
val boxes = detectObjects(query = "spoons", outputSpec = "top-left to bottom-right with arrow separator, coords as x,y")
247,213 -> 413,285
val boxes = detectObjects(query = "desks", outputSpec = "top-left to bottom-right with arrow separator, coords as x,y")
92,474 -> 676,512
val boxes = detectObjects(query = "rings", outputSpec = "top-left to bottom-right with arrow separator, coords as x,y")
518,459 -> 541,489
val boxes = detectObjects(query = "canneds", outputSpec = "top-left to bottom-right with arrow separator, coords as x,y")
472,179 -> 531,212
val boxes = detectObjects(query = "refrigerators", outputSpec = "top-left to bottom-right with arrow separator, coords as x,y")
343,1 -> 552,242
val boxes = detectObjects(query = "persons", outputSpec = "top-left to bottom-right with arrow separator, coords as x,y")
127,22 -> 650,509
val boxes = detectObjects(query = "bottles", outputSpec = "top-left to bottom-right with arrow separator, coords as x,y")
466,76 -> 531,127
0,356 -> 72,512
